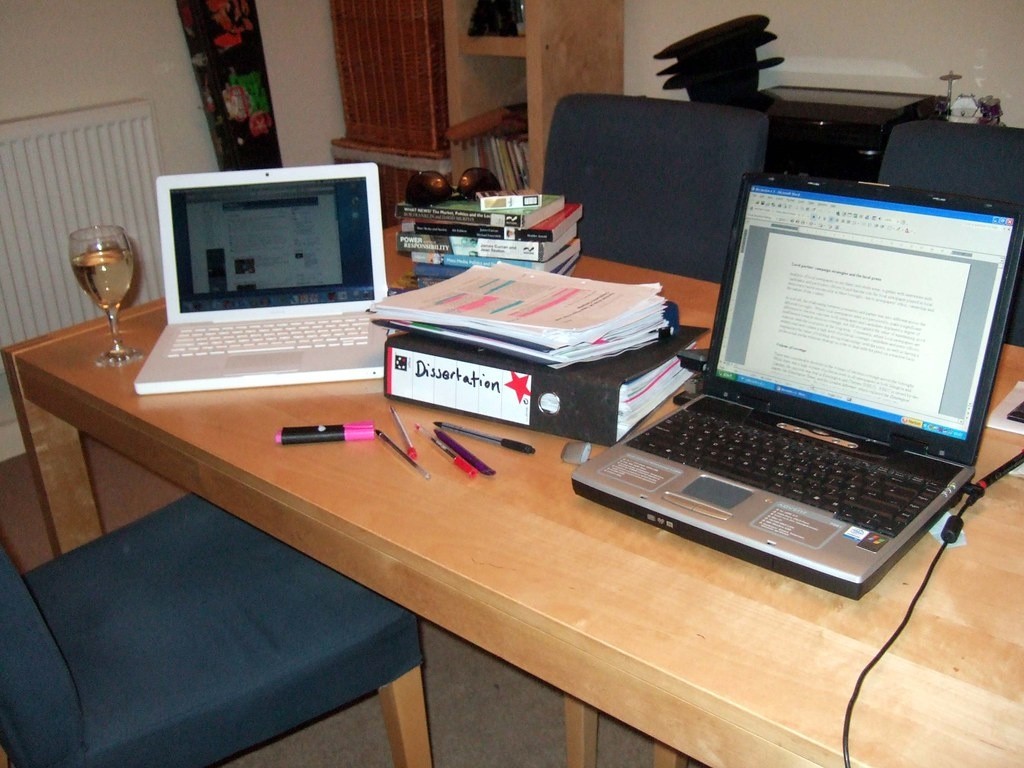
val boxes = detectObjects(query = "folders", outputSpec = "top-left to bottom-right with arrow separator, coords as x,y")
384,325 -> 713,446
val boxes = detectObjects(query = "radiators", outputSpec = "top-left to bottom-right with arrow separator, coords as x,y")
0,97 -> 167,425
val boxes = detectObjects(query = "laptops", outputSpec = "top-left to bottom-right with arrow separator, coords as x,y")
571,171 -> 1024,602
135,162 -> 391,395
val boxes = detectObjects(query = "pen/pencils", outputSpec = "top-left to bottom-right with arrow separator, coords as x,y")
375,404 -> 536,481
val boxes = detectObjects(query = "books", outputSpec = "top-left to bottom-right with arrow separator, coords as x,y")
395,187 -> 582,290
477,134 -> 531,190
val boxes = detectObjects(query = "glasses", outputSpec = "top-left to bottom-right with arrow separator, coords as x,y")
405,167 -> 503,208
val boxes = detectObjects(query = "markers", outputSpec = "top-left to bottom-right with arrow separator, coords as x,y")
274,420 -> 376,446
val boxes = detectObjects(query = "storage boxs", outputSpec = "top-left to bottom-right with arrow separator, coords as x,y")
330,1 -> 451,152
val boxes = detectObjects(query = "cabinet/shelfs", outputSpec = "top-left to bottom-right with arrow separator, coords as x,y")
330,0 -> 622,232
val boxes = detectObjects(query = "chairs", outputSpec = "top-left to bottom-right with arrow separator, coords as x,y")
1,489 -> 431,768
540,94 -> 770,768
656,124 -> 1024,768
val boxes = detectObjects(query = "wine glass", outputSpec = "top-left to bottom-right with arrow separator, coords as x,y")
69,225 -> 143,366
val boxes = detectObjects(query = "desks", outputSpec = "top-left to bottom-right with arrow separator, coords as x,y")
0,219 -> 1024,767
